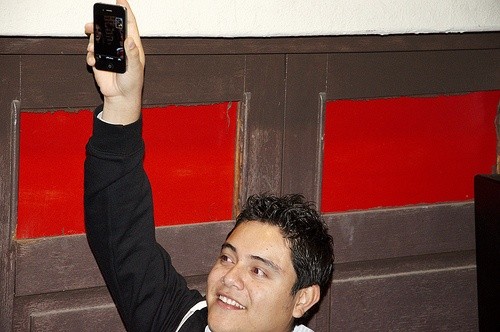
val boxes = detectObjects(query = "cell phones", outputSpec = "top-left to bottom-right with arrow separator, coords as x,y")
93,3 -> 128,73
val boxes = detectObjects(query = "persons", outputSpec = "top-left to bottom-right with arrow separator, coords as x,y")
84,0 -> 334,332
